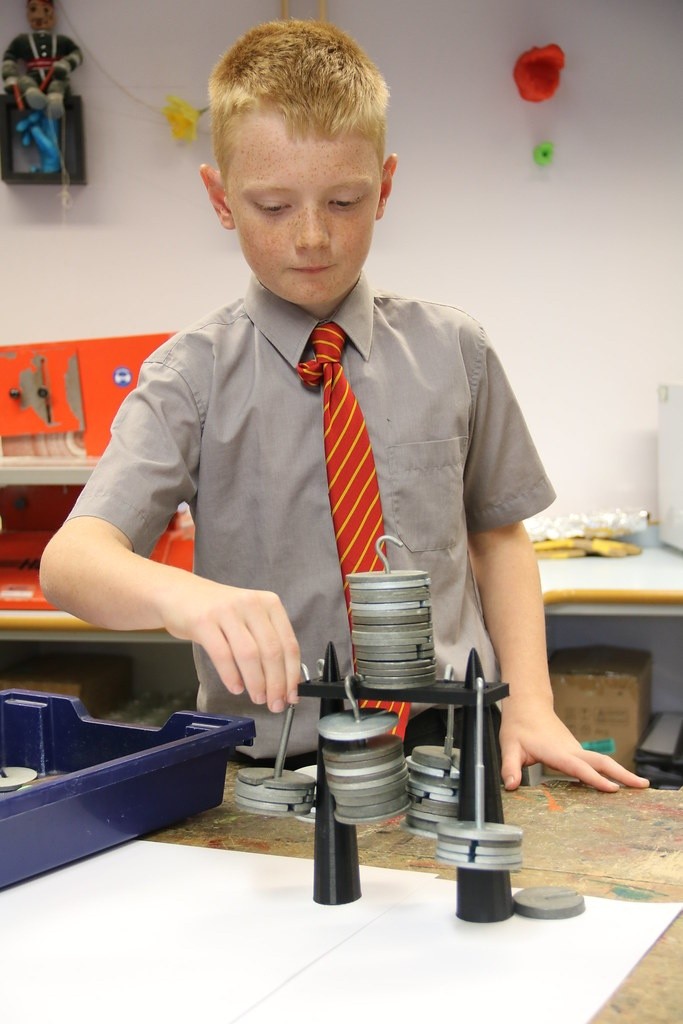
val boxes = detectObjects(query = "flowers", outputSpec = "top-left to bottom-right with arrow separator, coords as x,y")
163,93 -> 210,143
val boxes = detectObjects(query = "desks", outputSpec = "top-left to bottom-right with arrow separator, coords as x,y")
538,550 -> 683,618
0,758 -> 683,1024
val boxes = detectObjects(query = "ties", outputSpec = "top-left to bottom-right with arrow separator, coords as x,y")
294,321 -> 409,745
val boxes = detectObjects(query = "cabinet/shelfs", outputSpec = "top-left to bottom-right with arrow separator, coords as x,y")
0,458 -> 195,644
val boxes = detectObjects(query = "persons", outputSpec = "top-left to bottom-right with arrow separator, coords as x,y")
40,20 -> 651,793
1,0 -> 84,119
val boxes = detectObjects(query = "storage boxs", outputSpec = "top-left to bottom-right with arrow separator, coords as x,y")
0,653 -> 136,717
0,689 -> 258,889
545,647 -> 653,776
